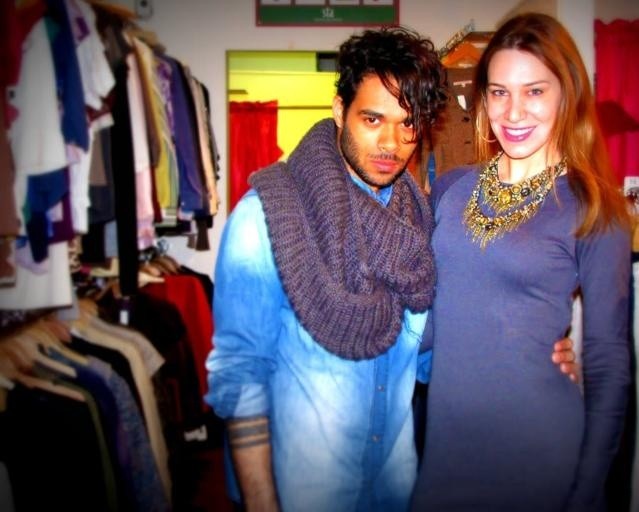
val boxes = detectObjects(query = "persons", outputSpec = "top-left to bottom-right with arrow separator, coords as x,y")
418,10 -> 631,512
198,23 -> 580,512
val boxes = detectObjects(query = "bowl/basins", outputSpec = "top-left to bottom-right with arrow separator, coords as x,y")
462,150 -> 568,248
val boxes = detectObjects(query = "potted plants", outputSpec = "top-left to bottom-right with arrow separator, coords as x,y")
256,2 -> 400,27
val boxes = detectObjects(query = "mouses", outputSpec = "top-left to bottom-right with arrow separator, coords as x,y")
1,1 -> 213,60
1,249 -> 199,403
434,29 -> 483,68
